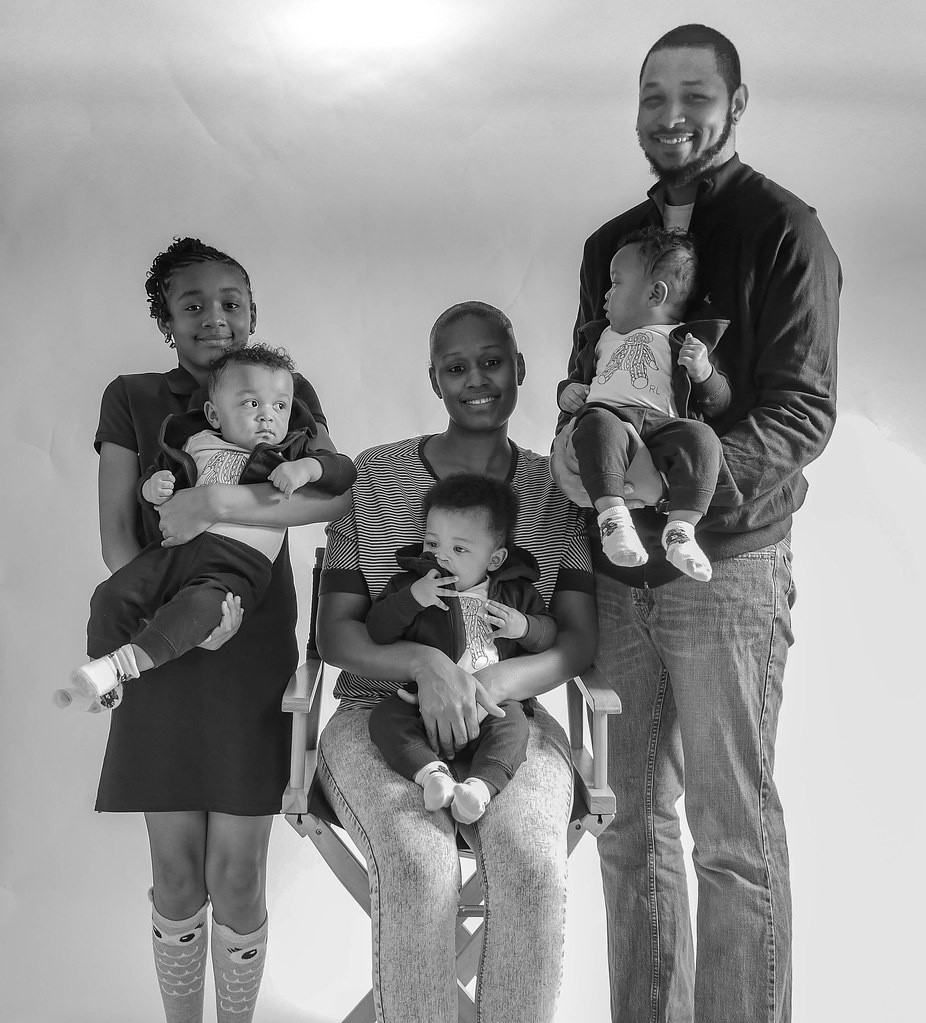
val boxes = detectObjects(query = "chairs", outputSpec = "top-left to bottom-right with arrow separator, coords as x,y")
281,546 -> 621,1023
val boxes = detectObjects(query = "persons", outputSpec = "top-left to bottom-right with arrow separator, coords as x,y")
550,24 -> 843,1023
365,473 -> 561,825
51,344 -> 358,716
95,236 -> 358,1023
314,300 -> 600,1023
556,226 -> 736,581
